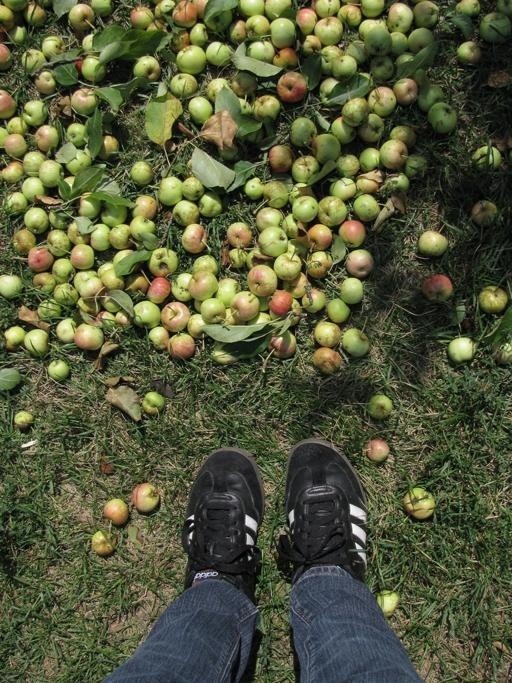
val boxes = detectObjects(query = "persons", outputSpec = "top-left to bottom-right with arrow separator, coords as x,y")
107,441 -> 422,683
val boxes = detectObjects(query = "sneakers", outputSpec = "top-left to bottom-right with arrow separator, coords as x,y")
286,439 -> 368,587
183,447 -> 263,606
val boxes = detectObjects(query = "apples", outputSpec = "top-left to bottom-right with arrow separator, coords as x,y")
0,0 -> 512,616
